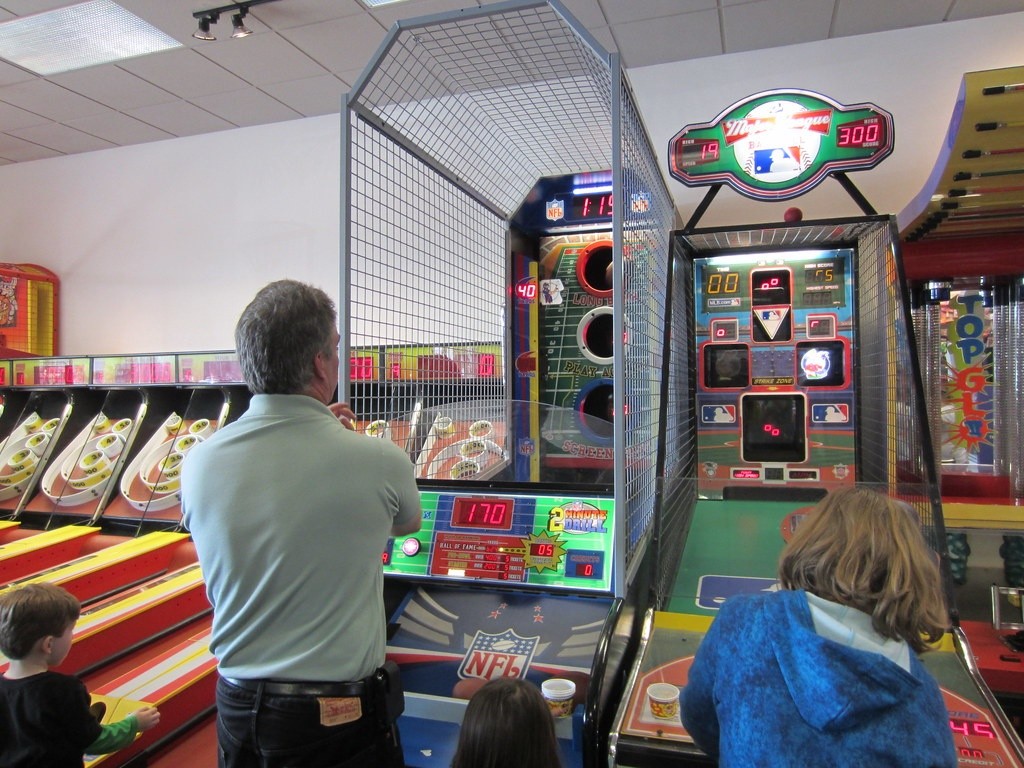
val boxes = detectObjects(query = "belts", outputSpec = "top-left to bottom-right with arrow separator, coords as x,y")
225,674 -> 364,699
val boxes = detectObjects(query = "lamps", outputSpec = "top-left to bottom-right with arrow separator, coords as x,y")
191,1 -> 270,41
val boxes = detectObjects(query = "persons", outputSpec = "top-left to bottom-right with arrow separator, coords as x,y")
0,581 -> 161,768
447,676 -> 559,768
180,280 -> 423,768
678,486 -> 957,768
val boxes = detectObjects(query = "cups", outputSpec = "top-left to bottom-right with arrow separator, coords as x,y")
646,683 -> 679,719
542,678 -> 576,719
